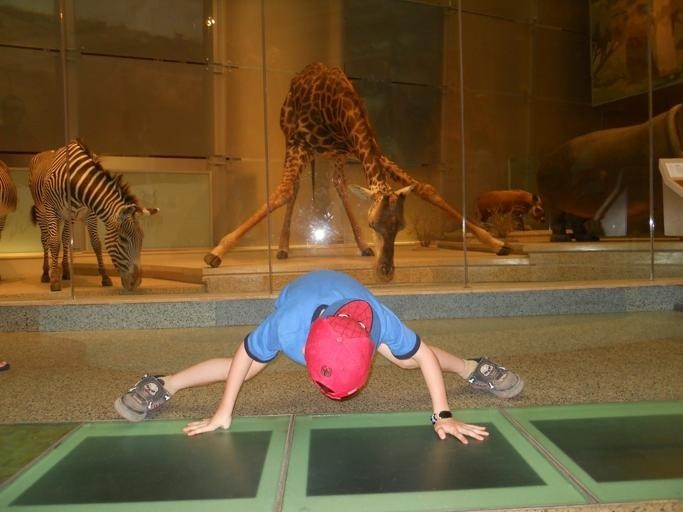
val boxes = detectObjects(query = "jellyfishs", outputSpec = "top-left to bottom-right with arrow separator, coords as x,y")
473,188 -> 546,234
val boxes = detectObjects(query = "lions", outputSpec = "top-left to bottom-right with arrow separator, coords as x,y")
27,139 -> 158,292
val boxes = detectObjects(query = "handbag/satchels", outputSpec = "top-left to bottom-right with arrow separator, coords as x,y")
430,409 -> 453,425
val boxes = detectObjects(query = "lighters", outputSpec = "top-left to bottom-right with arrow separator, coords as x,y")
202,61 -> 512,286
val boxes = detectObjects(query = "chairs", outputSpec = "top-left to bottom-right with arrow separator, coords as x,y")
461,354 -> 525,402
113,371 -> 173,424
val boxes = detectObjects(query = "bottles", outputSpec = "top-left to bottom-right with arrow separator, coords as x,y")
302,296 -> 382,404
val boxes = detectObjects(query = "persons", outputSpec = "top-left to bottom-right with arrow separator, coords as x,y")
114,269 -> 525,444
